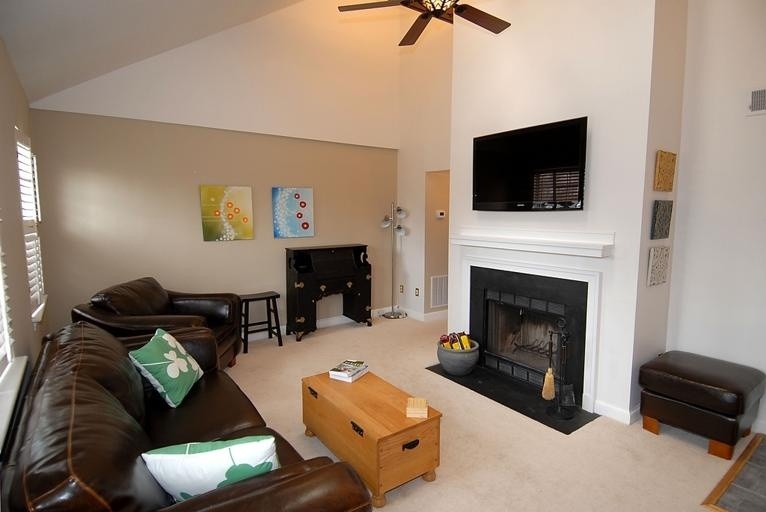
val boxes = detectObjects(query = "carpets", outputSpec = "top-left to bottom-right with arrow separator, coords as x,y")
701,432 -> 766,512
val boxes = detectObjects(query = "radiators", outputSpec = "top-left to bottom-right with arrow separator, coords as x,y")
430,275 -> 448,308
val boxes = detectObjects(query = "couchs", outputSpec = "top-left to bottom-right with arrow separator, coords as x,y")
7,320 -> 375,510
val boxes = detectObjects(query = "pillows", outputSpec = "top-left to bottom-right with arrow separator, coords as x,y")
128,328 -> 205,408
140,434 -> 282,504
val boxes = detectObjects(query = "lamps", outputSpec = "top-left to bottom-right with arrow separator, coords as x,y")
420,0 -> 459,18
380,201 -> 406,319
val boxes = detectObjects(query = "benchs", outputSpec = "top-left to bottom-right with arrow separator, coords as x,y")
637,349 -> 766,461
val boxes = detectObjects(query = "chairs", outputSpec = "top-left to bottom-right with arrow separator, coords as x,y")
70,276 -> 242,372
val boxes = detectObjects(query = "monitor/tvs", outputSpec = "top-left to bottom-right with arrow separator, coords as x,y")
472,116 -> 589,212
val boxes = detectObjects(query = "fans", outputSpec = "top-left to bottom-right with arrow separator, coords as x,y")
338,0 -> 510,46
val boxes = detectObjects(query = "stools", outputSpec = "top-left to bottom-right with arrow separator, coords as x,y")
239,290 -> 282,353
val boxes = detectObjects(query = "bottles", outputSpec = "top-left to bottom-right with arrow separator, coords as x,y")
542,367 -> 556,400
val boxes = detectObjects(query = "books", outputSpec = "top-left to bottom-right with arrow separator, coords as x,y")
329,359 -> 368,383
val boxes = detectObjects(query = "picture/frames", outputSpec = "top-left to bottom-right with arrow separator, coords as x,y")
646,245 -> 669,285
653,149 -> 676,193
650,199 -> 673,240
